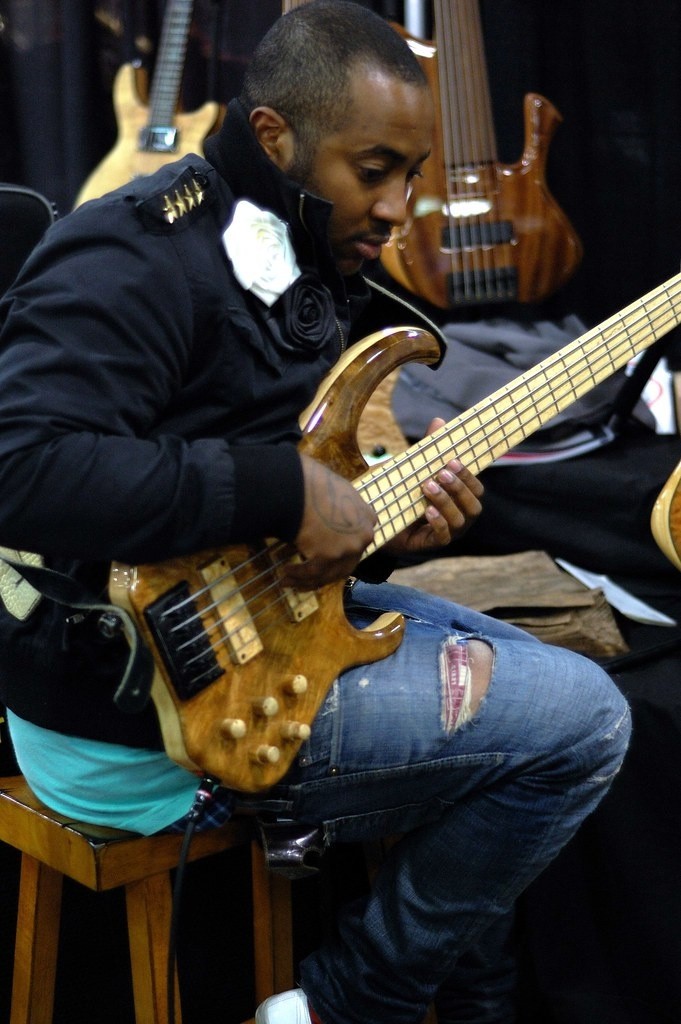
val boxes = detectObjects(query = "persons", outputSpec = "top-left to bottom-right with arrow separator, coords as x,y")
0,0 -> 633,1024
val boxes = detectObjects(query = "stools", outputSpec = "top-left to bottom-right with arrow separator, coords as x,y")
0,777 -> 294,1024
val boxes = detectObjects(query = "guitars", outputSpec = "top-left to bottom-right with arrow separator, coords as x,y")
107,271 -> 681,795
69,0 -> 227,214
380,0 -> 582,310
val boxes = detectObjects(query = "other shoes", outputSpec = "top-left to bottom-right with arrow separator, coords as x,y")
255,987 -> 323,1024
